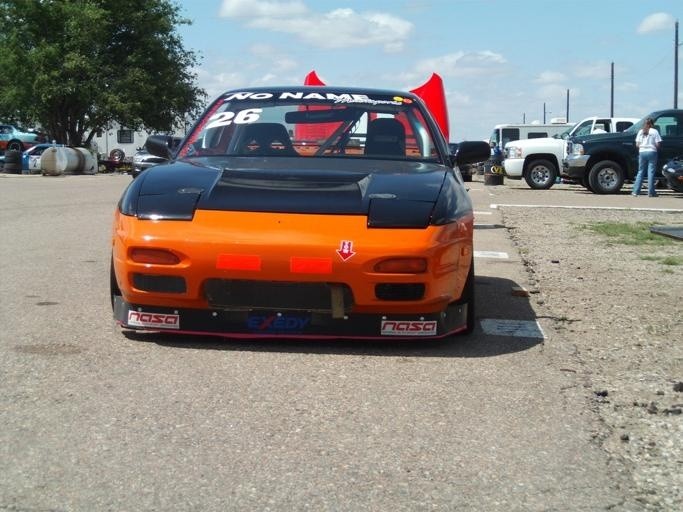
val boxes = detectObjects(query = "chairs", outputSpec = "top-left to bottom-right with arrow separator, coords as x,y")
225,123 -> 302,157
364,119 -> 406,157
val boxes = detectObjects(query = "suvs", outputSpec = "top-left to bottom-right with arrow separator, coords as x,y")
130,134 -> 184,180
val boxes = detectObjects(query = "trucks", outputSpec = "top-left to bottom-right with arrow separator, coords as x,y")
484,107 -> 683,191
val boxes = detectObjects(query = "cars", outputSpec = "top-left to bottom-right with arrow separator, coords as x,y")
660,153 -> 682,193
442,137 -> 478,185
0,122 -> 82,174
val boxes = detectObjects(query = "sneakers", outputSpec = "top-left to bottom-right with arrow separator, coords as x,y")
630,192 -> 658,197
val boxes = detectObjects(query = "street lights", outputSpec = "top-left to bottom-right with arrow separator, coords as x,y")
543,110 -> 552,124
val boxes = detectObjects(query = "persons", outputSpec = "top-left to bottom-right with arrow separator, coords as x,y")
491,142 -> 503,164
630,118 -> 663,197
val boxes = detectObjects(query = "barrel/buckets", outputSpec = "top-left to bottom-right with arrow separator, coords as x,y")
449,144 -> 473,182
484,155 -> 503,186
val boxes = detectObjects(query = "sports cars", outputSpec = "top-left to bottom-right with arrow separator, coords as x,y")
104,69 -> 490,348
